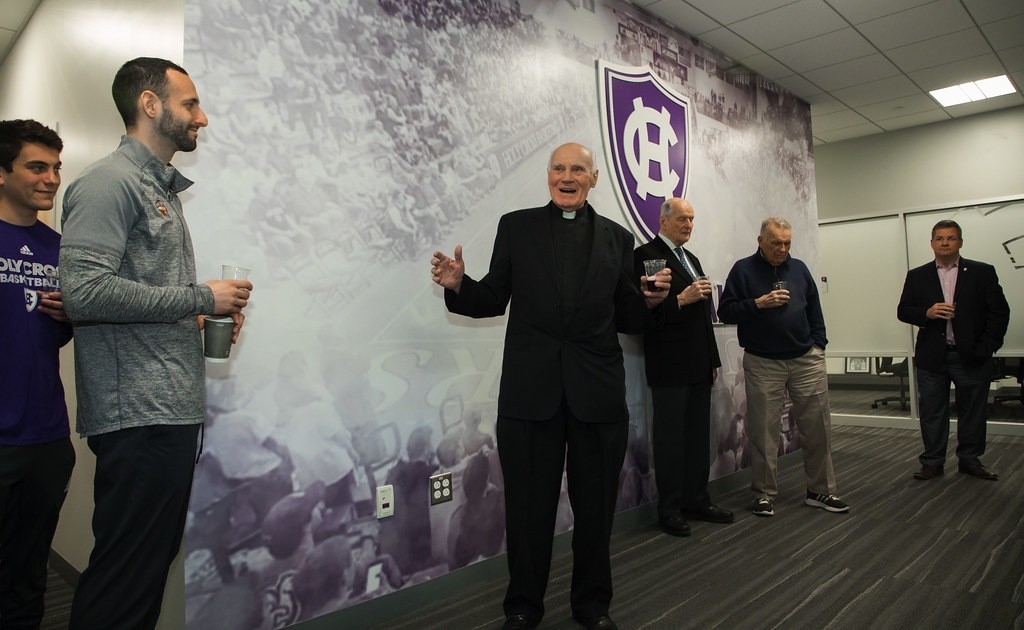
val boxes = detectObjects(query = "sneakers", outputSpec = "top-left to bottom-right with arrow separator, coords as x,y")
805,492 -> 850,512
751,497 -> 775,515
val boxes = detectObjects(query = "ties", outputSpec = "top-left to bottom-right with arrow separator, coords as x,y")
676,248 -> 696,282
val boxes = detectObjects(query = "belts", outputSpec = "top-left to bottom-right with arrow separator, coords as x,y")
946,345 -> 957,351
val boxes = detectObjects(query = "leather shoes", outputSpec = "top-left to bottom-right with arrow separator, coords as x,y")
502,609 -> 535,630
914,465 -> 943,480
958,463 -> 998,478
661,516 -> 690,536
680,504 -> 734,522
586,615 -> 618,630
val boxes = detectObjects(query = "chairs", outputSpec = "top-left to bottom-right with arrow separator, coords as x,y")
992,357 -> 1024,415
872,357 -> 911,411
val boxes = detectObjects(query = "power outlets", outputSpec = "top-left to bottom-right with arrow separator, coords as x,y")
429,472 -> 454,505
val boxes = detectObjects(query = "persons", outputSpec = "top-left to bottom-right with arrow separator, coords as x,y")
184,0 -> 820,630
716,217 -> 850,515
0,119 -> 76,630
897,219 -> 1010,478
430,143 -> 672,630
60,57 -> 253,630
634,197 -> 735,537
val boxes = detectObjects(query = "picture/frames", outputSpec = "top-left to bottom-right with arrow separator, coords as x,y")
845,357 -> 872,375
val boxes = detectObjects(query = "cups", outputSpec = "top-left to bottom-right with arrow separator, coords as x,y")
222,264 -> 252,290
643,259 -> 668,293
773,281 -> 788,296
696,276 -> 711,281
945,303 -> 956,318
203,315 -> 236,363
43,270 -> 62,297
